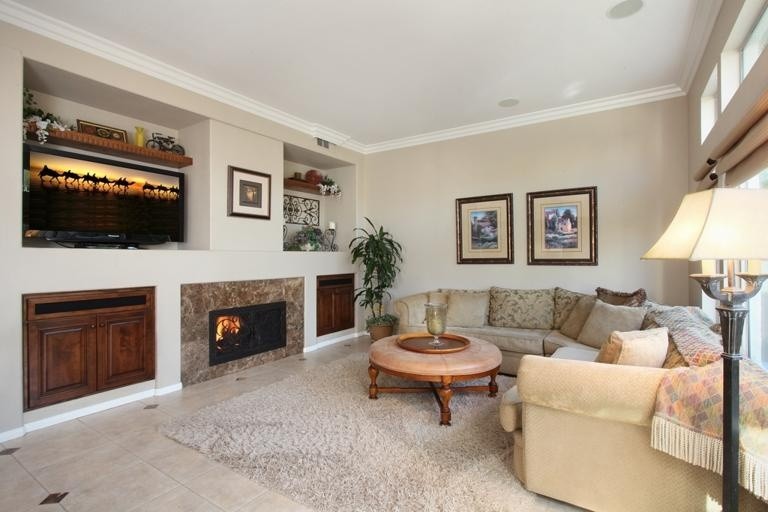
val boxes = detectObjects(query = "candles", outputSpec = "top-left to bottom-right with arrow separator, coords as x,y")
324,220 -> 337,231
283,217 -> 287,226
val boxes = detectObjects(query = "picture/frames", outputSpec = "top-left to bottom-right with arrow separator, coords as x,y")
455,193 -> 514,264
526,186 -> 598,266
227,165 -> 271,220
76,119 -> 128,144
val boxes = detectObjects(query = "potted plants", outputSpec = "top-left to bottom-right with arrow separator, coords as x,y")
348,217 -> 403,344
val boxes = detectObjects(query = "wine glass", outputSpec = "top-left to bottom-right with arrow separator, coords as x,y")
424,303 -> 447,345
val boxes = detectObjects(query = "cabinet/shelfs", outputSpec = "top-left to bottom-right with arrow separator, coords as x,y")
22,286 -> 155,413
316,273 -> 355,337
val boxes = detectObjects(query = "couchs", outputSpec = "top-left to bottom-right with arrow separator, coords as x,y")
392,286 -> 768,512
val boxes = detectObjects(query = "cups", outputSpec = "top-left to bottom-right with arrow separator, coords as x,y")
294,172 -> 301,179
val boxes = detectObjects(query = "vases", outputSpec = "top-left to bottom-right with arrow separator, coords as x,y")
299,240 -> 321,251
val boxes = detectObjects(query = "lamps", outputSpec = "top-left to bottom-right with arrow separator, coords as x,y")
640,187 -> 768,512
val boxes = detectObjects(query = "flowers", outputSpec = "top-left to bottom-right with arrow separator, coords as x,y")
291,222 -> 324,250
315,174 -> 344,202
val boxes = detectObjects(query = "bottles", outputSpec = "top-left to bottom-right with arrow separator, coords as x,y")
305,170 -> 323,183
134,126 -> 144,147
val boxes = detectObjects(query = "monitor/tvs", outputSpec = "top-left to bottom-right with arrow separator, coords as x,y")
22,144 -> 184,249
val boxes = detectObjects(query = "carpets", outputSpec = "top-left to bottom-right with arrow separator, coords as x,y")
157,352 -> 593,512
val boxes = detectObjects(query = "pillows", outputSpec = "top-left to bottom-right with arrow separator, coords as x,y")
444,293 -> 489,327
488,287 -> 555,329
578,299 -> 647,349
595,286 -> 647,307
612,332 -> 669,368
593,327 -> 669,368
560,296 -> 596,340
551,286 -> 588,329
